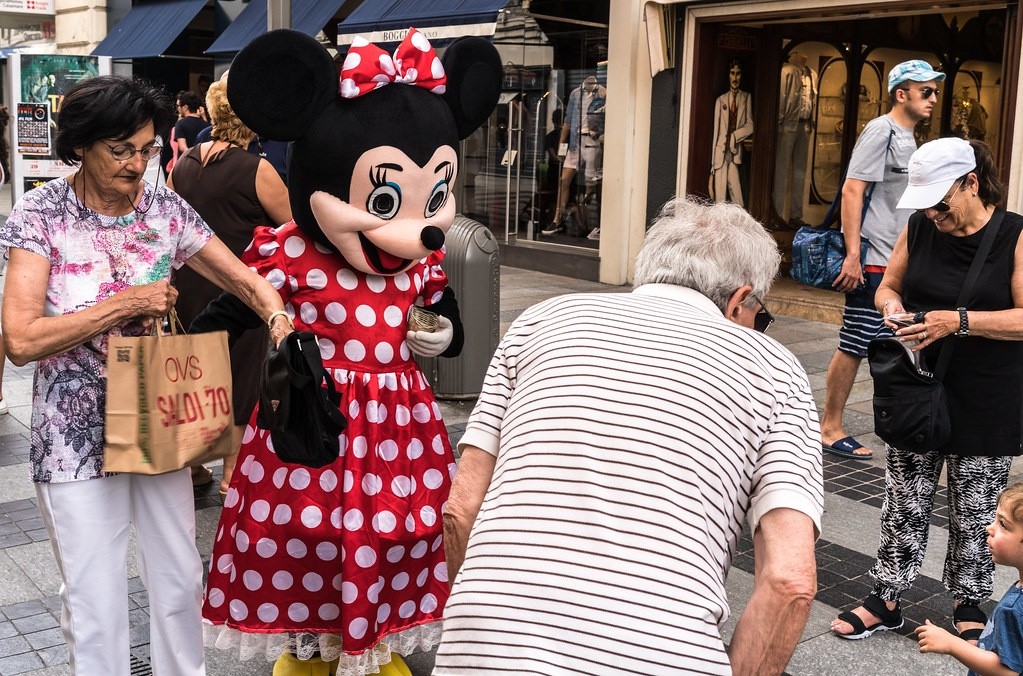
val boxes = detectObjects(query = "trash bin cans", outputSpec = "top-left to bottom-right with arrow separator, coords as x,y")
412,213 -> 500,400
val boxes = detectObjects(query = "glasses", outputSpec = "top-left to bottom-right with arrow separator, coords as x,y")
98,138 -> 163,161
176,103 -> 182,108
915,176 -> 966,212
750,291 -> 776,333
904,88 -> 939,99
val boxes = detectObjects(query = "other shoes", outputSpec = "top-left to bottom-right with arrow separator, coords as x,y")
542,222 -> 563,234
0,398 -> 9,415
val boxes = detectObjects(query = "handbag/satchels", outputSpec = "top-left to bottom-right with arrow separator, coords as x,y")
789,225 -> 869,292
260,331 -> 349,468
108,306 -> 233,475
867,338 -> 950,454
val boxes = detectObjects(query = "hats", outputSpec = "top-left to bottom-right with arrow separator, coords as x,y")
896,136 -> 976,209
887,60 -> 946,93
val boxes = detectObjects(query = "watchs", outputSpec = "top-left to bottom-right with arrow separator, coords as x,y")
954,307 -> 970,337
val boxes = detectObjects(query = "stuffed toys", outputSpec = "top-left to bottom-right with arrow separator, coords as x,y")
202,28 -> 506,676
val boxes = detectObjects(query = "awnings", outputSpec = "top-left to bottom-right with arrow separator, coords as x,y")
90,0 -> 209,64
203,0 -> 346,55
337,0 -> 509,55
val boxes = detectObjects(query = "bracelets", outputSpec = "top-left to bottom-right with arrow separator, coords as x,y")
885,300 -> 905,312
268,311 -> 293,329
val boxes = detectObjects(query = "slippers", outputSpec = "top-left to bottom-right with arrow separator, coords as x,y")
192,466 -> 213,485
219,489 -> 228,504
822,436 -> 873,460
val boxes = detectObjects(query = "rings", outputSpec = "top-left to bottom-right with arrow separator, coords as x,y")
923,332 -> 926,338
914,311 -> 927,323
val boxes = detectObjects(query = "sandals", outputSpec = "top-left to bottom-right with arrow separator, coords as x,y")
835,594 -> 904,640
952,603 -> 988,641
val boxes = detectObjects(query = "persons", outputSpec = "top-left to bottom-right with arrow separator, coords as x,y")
167,81 -> 293,504
774,54 -> 819,226
431,201 -> 824,676
830,137 -> 1023,647
166,91 -> 209,173
820,60 -> 945,458
913,482 -> 1023,676
541,74 -> 607,243
1,76 -> 295,676
